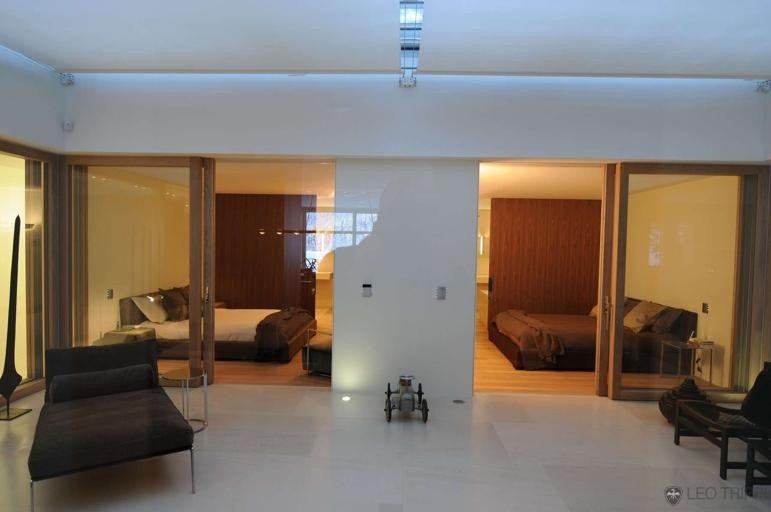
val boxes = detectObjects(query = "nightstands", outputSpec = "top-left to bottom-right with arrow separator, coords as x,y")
215,302 -> 226,309
103,328 -> 156,346
660,340 -> 712,386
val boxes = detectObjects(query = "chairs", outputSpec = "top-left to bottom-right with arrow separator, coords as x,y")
676,360 -> 771,497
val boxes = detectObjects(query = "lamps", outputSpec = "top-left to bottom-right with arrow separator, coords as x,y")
399,0 -> 425,88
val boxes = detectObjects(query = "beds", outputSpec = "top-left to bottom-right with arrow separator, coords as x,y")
120,286 -> 318,364
489,296 -> 698,371
28,339 -> 195,512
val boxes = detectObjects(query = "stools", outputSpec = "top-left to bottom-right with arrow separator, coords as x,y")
161,367 -> 208,434
302,329 -> 332,376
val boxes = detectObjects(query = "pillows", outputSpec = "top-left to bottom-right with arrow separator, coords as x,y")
131,284 -> 204,324
48,364 -> 155,403
589,297 -> 683,335
